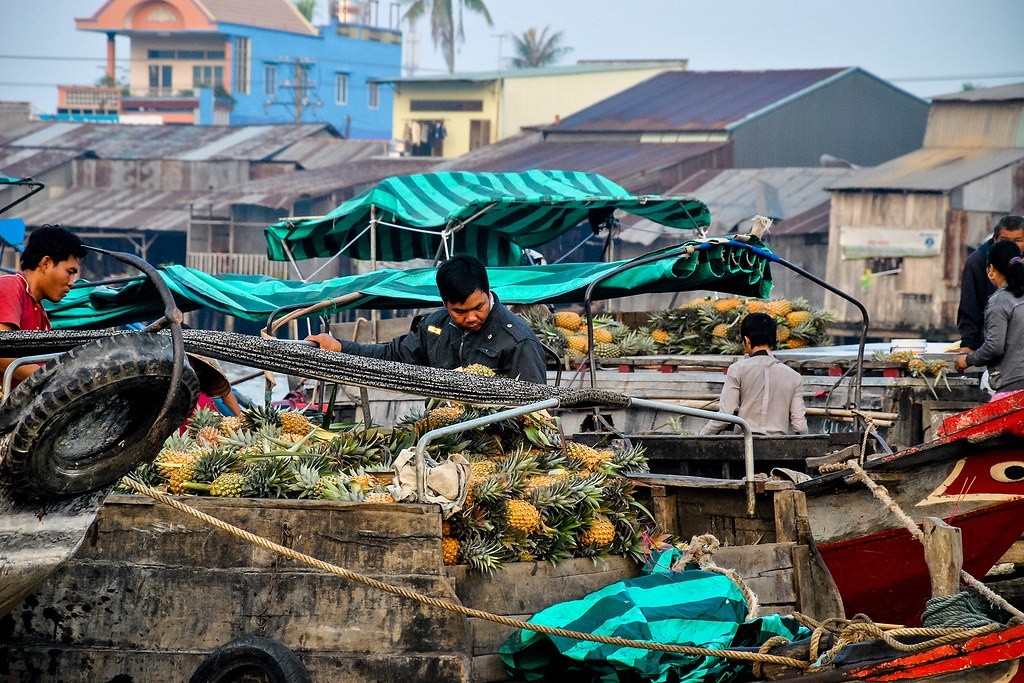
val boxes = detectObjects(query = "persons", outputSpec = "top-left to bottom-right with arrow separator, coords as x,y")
945,238 -> 1024,400
945,216 -> 1024,355
432,121 -> 447,158
696,312 -> 811,435
303,254 -> 546,410
211,390 -> 241,417
0,224 -> 89,396
175,351 -> 233,439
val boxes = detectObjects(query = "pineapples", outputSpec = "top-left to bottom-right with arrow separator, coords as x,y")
135,363 -> 649,579
515,297 -> 837,361
871,350 -> 952,402
